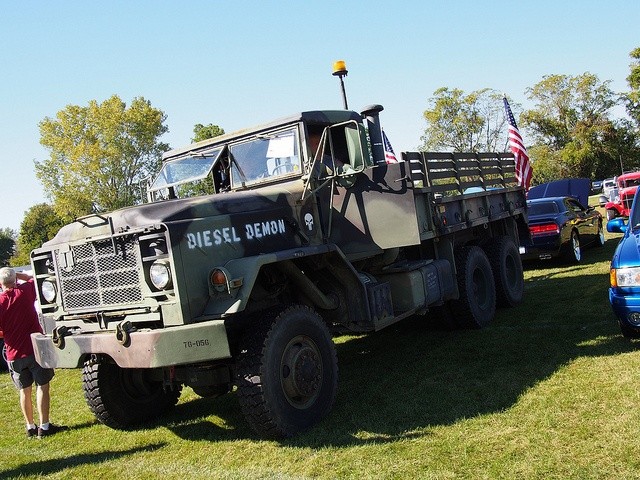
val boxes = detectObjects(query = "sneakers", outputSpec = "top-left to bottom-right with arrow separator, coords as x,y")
38,423 -> 68,436
28,423 -> 37,436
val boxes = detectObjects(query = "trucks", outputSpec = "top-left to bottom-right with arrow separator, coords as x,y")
29,61 -> 532,438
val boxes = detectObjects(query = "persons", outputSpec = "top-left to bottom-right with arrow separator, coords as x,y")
308,132 -> 343,173
1,266 -> 68,437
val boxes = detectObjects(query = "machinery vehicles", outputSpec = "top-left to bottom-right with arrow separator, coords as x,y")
605,172 -> 640,220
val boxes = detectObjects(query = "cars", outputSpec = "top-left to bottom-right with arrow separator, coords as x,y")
518,196 -> 604,264
608,186 -> 640,338
591,177 -> 612,195
